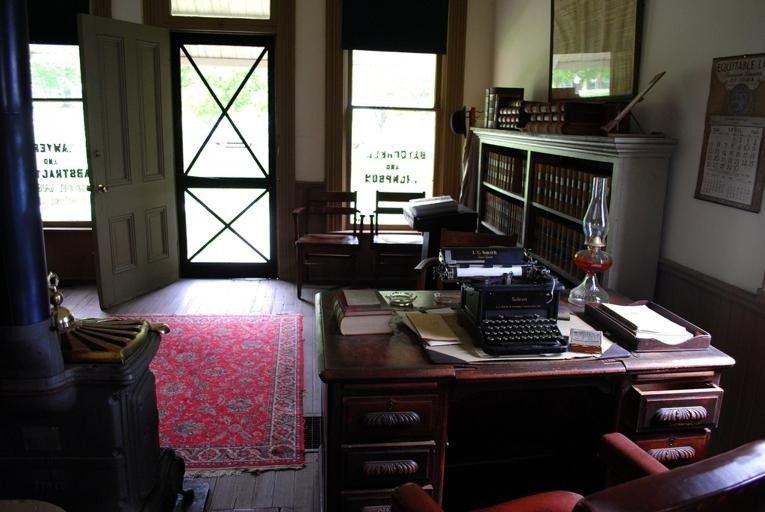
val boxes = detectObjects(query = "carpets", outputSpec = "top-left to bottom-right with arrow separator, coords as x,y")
109,313 -> 305,474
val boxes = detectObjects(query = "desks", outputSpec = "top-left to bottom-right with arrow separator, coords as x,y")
313,288 -> 737,512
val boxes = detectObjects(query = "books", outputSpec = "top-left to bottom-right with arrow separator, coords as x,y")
601,302 -> 694,346
484,87 -> 606,136
401,313 -> 462,348
408,195 -> 459,217
482,149 -> 611,284
333,289 -> 394,336
569,327 -> 602,353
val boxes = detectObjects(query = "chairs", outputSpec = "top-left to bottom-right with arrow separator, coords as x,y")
292,191 -> 361,298
369,191 -> 430,286
414,228 -> 517,288
395,435 -> 765,511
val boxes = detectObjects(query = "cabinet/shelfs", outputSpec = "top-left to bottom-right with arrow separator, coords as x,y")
471,126 -> 677,302
